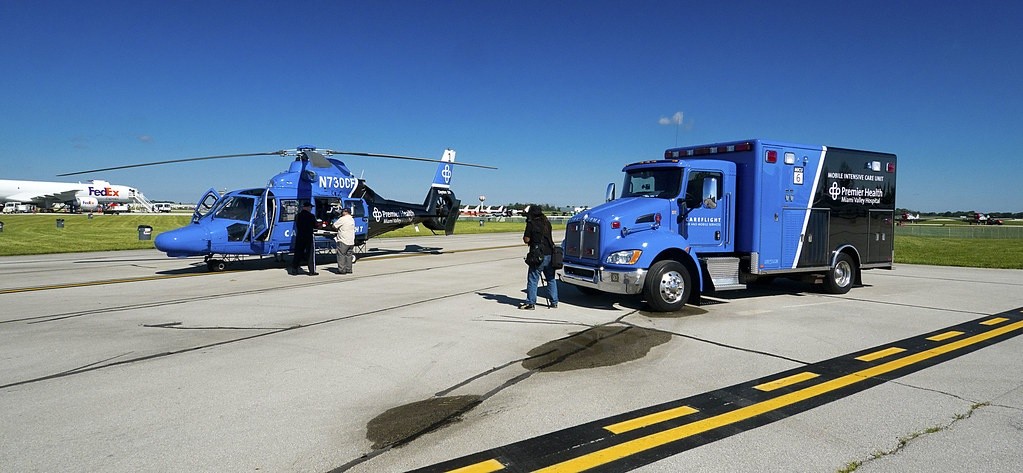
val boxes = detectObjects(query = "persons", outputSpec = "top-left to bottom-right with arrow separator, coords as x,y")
332,207 -> 355,274
902,210 -> 907,219
292,201 -> 323,276
518,204 -> 558,310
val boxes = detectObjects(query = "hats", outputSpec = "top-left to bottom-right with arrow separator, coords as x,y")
528,206 -> 542,215
341,208 -> 351,213
302,200 -> 310,207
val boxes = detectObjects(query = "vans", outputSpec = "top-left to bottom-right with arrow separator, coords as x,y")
152,203 -> 172,213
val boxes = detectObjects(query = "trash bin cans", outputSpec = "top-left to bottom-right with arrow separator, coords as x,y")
0,221 -> 4,232
87,213 -> 93,219
56,218 -> 65,228
479,219 -> 485,226
137,224 -> 152,240
488,218 -> 491,222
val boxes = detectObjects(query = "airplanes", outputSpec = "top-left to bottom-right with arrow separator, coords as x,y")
0,179 -> 158,214
457,205 -> 534,218
894,213 -> 927,225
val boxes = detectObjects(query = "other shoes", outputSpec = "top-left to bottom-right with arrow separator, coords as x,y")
336,271 -> 343,274
309,272 -> 319,275
291,264 -> 297,274
551,303 -> 557,308
526,305 -> 535,310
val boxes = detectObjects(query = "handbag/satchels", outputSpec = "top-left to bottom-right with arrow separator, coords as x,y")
525,253 -> 544,269
552,246 -> 563,268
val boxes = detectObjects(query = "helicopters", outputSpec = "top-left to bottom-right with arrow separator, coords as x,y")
57,145 -> 499,274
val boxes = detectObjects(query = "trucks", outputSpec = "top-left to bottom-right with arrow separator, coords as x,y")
558,139 -> 897,313
2,201 -> 22,214
